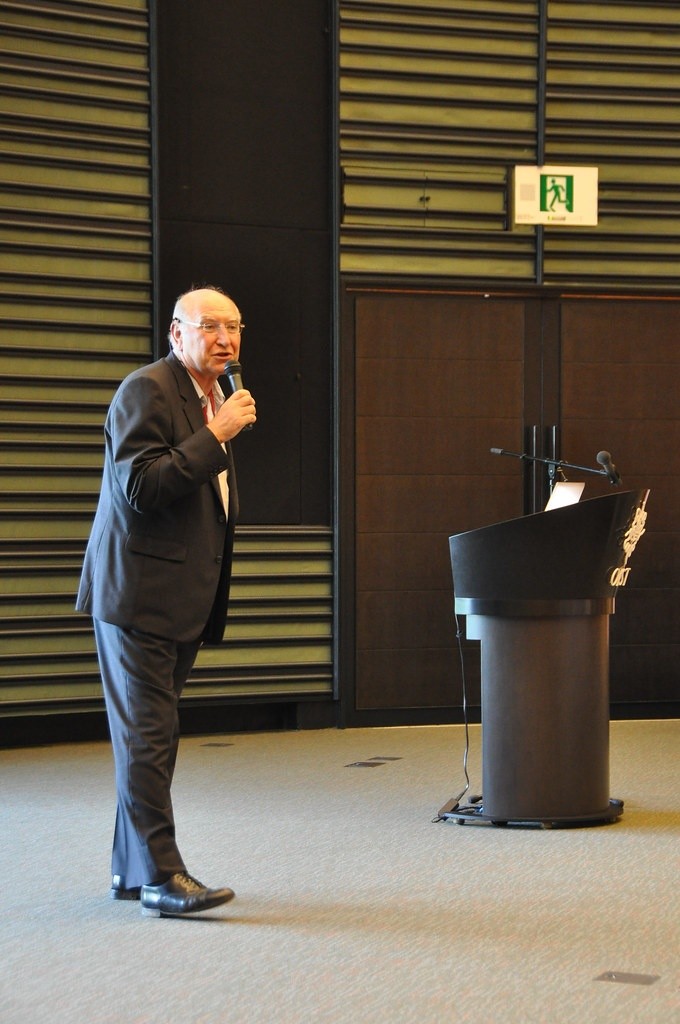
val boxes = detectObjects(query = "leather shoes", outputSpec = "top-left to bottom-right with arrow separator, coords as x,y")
141,874 -> 234,917
110,873 -> 141,900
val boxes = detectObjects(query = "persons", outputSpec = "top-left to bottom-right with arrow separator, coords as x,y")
72,287 -> 259,914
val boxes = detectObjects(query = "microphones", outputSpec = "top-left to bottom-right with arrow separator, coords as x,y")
597,451 -> 622,486
224,360 -> 253,431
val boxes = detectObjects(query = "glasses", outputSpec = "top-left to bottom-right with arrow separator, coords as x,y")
173,317 -> 245,335
